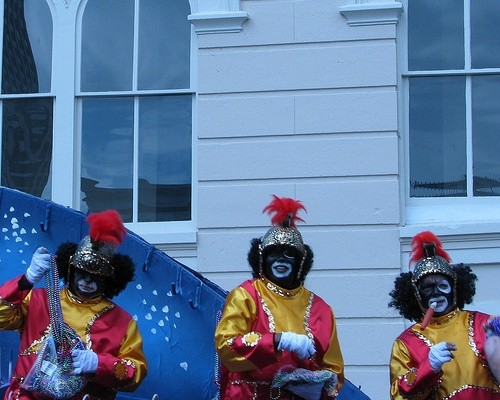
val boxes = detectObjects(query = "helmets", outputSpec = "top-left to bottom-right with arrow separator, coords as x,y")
388,232 -> 477,322
247,194 -> 314,280
56,210 -> 135,299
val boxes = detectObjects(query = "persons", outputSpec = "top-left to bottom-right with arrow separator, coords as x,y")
389,230 -> 500,400
0,210 -> 149,400
213,195 -> 345,400
